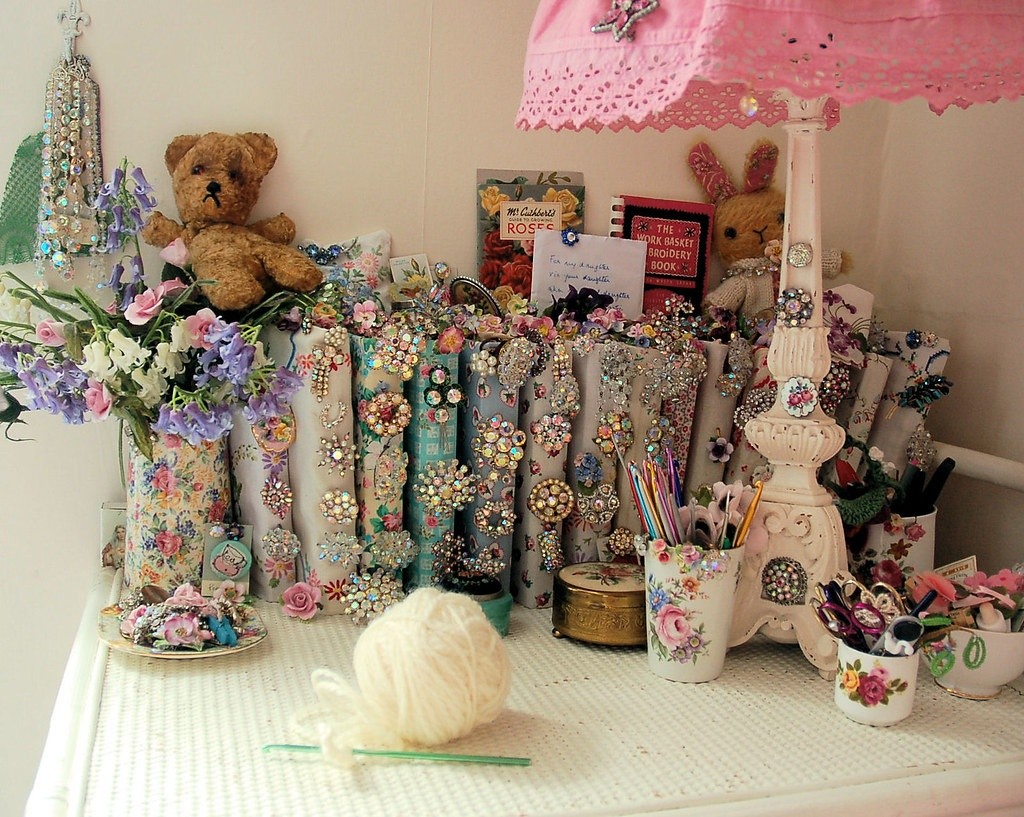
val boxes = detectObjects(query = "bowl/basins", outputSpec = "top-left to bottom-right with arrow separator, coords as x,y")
921,625 -> 1024,699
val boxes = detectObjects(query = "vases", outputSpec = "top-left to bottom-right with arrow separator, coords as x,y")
123,437 -> 232,593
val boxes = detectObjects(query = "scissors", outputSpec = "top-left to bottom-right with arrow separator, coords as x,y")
810,569 -> 906,654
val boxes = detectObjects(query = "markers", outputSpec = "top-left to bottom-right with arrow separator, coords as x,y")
919,453 -> 958,511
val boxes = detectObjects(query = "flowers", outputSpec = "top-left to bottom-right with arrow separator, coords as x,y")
212,580 -> 245,604
121,604 -> 147,638
312,271 -> 658,348
1,156 -> 320,460
164,583 -> 209,608
279,581 -> 324,624
152,614 -> 213,652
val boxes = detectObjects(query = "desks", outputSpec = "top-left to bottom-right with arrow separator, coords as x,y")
27,432 -> 1024,817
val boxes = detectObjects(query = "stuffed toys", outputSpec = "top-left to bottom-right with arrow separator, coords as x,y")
687,138 -> 848,324
140,131 -> 320,313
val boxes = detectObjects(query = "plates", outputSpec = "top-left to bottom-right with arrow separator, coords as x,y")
98,602 -> 267,658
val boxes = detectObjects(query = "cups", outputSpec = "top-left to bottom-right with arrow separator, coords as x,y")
835,639 -> 920,726
848,505 -> 937,596
644,540 -> 745,682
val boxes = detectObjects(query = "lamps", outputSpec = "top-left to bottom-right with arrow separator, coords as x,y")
516,0 -> 1024,679
